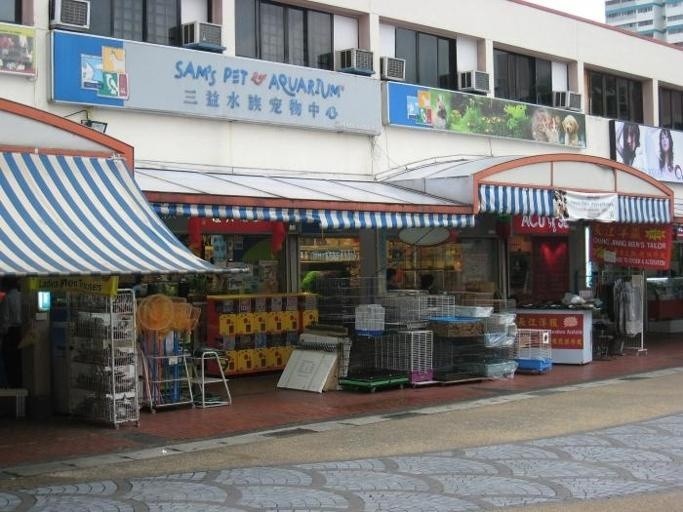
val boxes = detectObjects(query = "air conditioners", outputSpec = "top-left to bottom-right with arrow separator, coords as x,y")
49,0 -> 90,31
340,49 -> 373,70
380,56 -> 405,82
181,21 -> 221,46
460,70 -> 490,93
553,91 -> 582,111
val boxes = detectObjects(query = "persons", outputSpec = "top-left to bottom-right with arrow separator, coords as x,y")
2,282 -> 22,388
615,123 -> 646,171
656,128 -> 678,176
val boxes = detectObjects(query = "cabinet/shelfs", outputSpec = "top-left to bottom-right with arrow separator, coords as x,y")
49,288 -> 140,429
